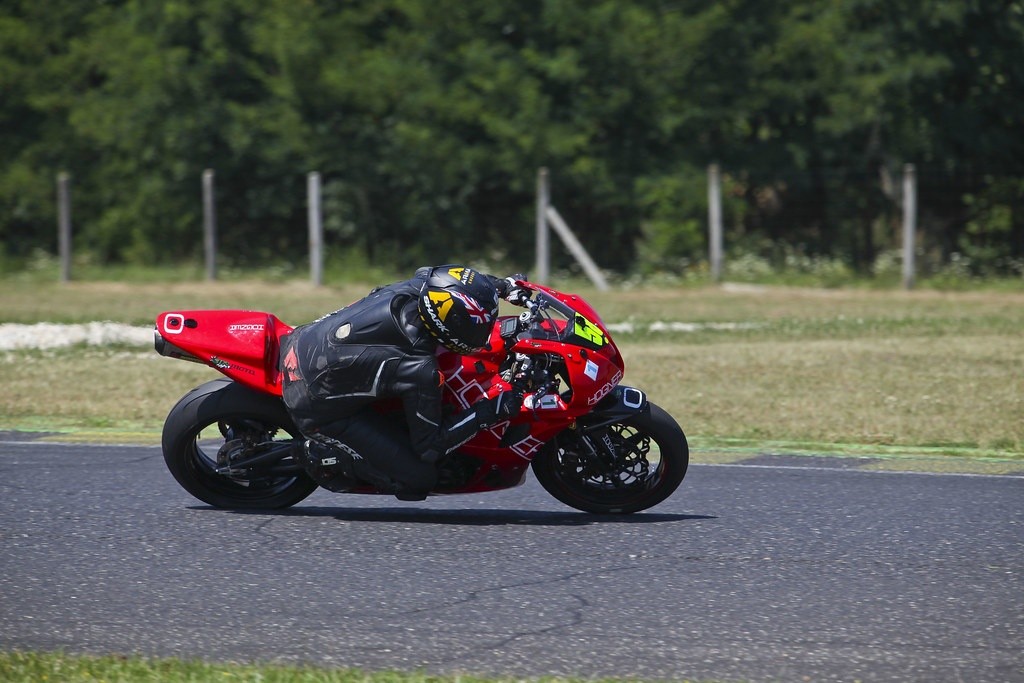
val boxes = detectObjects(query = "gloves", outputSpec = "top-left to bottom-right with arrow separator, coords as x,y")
488,389 -> 524,417
499,274 -> 533,306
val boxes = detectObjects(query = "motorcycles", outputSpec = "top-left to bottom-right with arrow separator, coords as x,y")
152,277 -> 690,515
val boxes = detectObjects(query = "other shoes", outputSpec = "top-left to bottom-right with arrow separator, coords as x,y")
290,439 -> 333,482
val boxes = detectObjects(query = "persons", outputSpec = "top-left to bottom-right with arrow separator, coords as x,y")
282,266 -> 532,496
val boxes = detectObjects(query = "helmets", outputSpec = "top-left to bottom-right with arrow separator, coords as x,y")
416,264 -> 499,356
